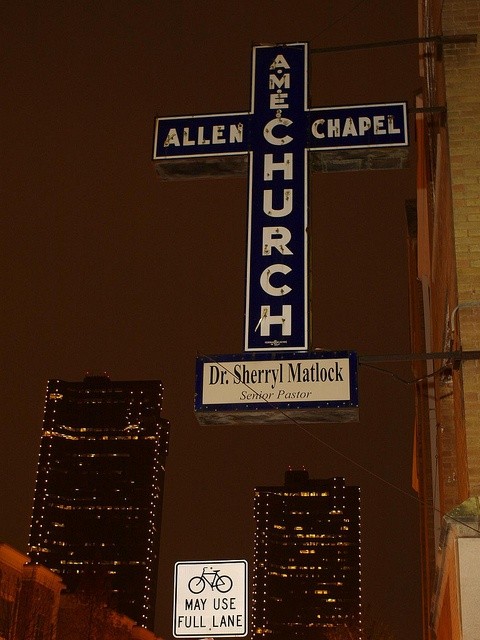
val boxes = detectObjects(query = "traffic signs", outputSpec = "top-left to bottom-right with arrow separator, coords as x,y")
171,559 -> 247,637
193,350 -> 356,409
